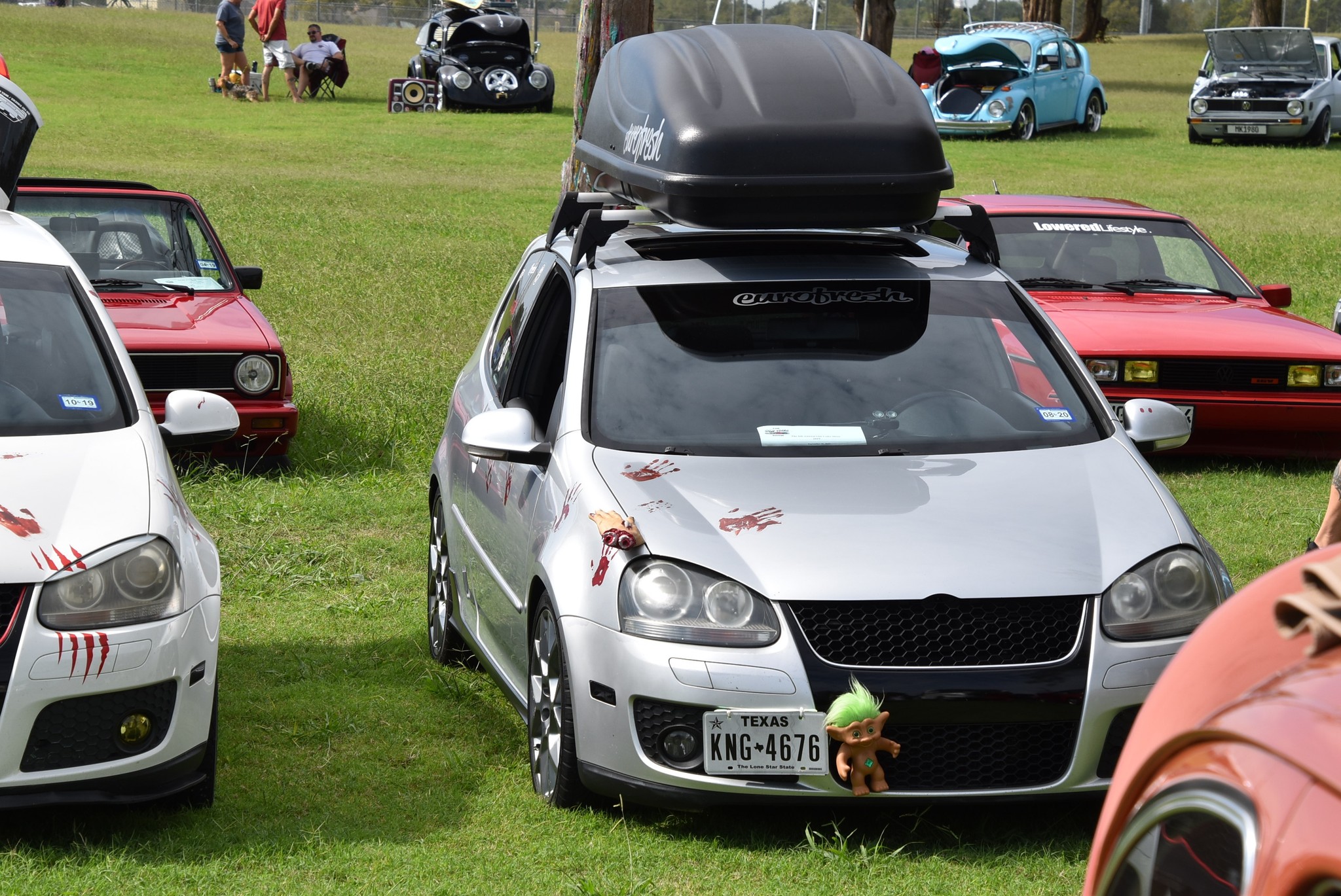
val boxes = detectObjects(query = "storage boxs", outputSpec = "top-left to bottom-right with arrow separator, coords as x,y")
231,70 -> 262,94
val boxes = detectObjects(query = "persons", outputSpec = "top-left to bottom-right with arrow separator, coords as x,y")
215,0 -> 251,98
247,0 -> 306,103
290,24 -> 344,99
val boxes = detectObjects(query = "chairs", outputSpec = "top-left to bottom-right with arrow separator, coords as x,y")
286,33 -> 347,99
1070,254 -> 1123,289
85,221 -> 171,270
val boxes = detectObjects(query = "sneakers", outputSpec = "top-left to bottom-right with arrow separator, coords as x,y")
306,62 -> 317,71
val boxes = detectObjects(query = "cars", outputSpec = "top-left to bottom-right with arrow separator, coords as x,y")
1186,25 -> 1341,150
10,177 -> 300,482
1080,541 -> 1341,896
407,0 -> 557,118
1,54 -> 222,837
907,25 -> 1108,142
426,22 -> 1241,841
864,178 -> 1341,477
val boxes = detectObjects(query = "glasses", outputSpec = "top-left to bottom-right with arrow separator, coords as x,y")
307,31 -> 320,35
